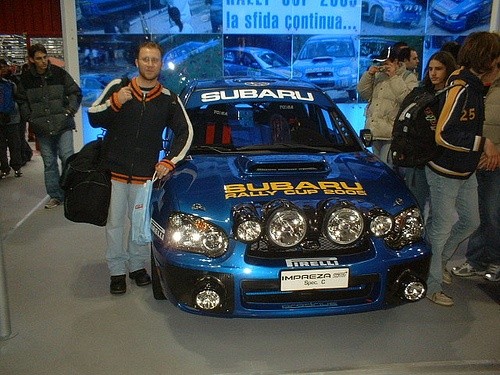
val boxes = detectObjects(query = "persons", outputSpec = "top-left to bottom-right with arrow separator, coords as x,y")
20,43 -> 84,209
360,30 -> 500,306
86,43 -> 195,294
0,58 -> 33,180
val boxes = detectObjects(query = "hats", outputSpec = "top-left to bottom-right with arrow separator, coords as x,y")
372,47 -> 398,63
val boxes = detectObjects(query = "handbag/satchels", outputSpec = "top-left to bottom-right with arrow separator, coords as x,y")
58,137 -> 111,226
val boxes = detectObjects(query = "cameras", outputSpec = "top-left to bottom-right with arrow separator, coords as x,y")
376,66 -> 387,72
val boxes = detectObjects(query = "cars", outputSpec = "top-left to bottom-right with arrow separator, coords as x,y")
362,0 -> 424,29
79,74 -> 106,107
429,0 -> 493,33
149,75 -> 434,321
293,34 -> 359,101
161,38 -> 222,82
224,46 -> 310,81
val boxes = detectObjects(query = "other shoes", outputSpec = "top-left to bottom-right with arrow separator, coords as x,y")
27,137 -> 37,142
15,168 -> 22,177
0,172 -> 10,179
45,198 -> 62,209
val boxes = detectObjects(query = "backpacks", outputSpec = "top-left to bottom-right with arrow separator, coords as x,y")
390,82 -> 469,167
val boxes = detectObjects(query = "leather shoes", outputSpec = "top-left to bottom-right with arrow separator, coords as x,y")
110,274 -> 126,293
129,268 -> 152,285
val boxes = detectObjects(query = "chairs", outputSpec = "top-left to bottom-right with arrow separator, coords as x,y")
264,102 -> 309,147
193,103 -> 231,149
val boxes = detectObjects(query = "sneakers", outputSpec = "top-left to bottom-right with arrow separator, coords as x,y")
452,262 -> 485,276
442,261 -> 451,284
426,291 -> 454,305
485,263 -> 500,281
